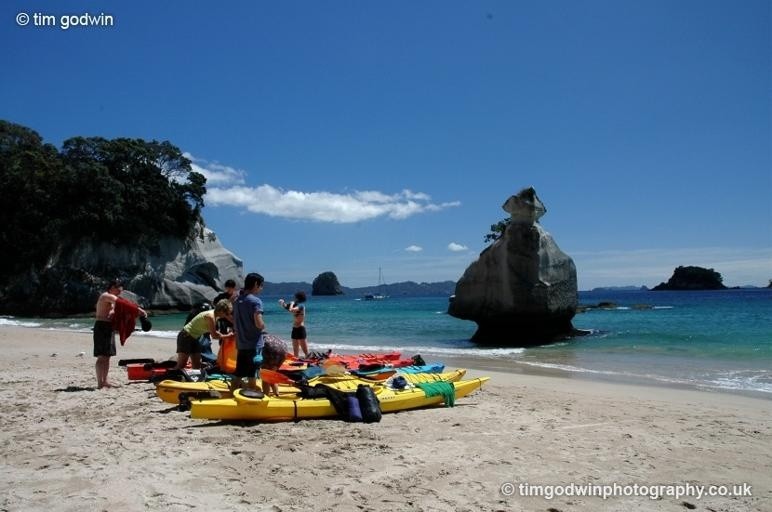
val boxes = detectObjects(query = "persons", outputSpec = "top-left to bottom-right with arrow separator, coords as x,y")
94,281 -> 148,389
278,292 -> 309,358
184,303 -> 211,339
260,331 -> 287,396
213,279 -> 240,345
229,273 -> 265,397
176,298 -> 236,369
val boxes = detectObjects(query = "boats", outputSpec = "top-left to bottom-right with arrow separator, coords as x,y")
361,292 -> 390,300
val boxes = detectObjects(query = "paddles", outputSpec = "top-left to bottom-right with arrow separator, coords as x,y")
259,368 -> 302,385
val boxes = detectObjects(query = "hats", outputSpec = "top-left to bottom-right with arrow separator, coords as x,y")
138,315 -> 151,331
